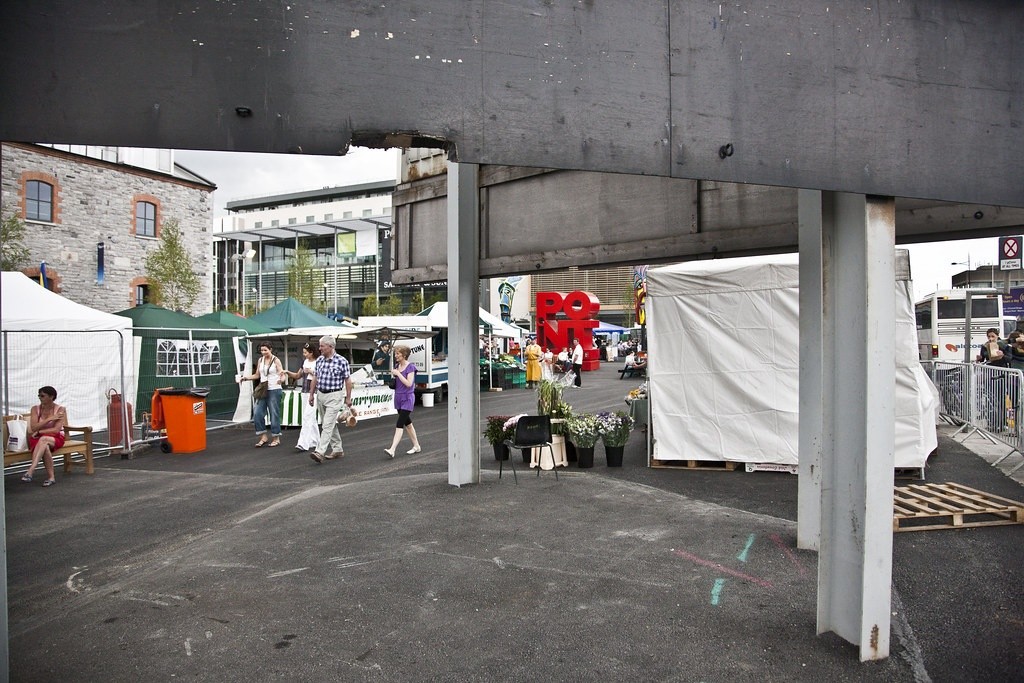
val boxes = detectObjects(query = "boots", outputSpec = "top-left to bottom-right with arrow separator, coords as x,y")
525,382 -> 532,389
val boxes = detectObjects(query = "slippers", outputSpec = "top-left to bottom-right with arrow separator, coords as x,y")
22,474 -> 34,482
42,479 -> 56,487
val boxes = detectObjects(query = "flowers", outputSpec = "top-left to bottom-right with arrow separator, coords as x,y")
567,414 -> 600,448
596,412 -> 636,448
503,413 -> 527,433
483,416 -> 511,443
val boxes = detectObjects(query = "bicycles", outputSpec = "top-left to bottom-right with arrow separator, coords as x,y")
940,360 -> 1010,433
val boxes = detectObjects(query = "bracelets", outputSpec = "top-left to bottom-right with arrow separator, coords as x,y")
37,429 -> 40,434
244,377 -> 246,381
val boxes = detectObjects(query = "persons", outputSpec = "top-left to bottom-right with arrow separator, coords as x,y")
373,340 -> 395,367
594,335 -> 647,379
278,342 -> 321,450
384,345 -> 422,459
524,336 -> 584,391
308,334 -> 352,463
977,328 -> 1024,368
238,340 -> 286,446
20,386 -> 65,486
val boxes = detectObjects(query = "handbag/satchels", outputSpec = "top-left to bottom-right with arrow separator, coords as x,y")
389,364 -> 399,389
253,381 -> 268,399
6,413 -> 29,452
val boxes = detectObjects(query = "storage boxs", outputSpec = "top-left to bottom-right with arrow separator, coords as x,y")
493,364 -> 527,390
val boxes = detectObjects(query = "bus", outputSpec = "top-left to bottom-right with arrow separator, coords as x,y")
913,288 -> 1005,371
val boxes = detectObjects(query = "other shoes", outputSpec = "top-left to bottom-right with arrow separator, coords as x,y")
325,452 -> 344,459
407,447 -> 421,454
571,385 -> 580,389
268,440 -> 280,446
311,452 -> 323,463
255,439 -> 269,447
384,449 -> 394,458
295,446 -> 305,451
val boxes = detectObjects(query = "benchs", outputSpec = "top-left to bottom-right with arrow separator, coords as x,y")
3,412 -> 94,480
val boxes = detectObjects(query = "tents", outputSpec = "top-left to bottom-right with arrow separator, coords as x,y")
643,245 -> 940,481
592,319 -> 627,334
1,269 -> 530,438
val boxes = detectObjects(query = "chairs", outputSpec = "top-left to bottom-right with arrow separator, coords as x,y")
499,414 -> 559,485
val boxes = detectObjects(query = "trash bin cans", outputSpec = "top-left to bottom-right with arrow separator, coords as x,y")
150,386 -> 212,455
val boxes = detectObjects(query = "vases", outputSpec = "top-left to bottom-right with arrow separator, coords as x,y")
577,447 -> 595,468
606,446 -> 624,468
492,442 -> 509,460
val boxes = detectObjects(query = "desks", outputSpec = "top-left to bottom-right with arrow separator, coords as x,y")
631,399 -> 649,431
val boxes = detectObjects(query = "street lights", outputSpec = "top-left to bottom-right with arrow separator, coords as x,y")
951,253 -> 970,287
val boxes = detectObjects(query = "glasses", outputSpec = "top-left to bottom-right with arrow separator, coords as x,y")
38,395 -> 50,397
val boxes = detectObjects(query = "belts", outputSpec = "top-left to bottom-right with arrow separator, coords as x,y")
318,388 -> 342,393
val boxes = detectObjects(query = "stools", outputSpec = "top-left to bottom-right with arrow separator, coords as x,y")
530,434 -> 568,471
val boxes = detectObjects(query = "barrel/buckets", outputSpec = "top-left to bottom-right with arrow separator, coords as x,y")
421,394 -> 434,407
